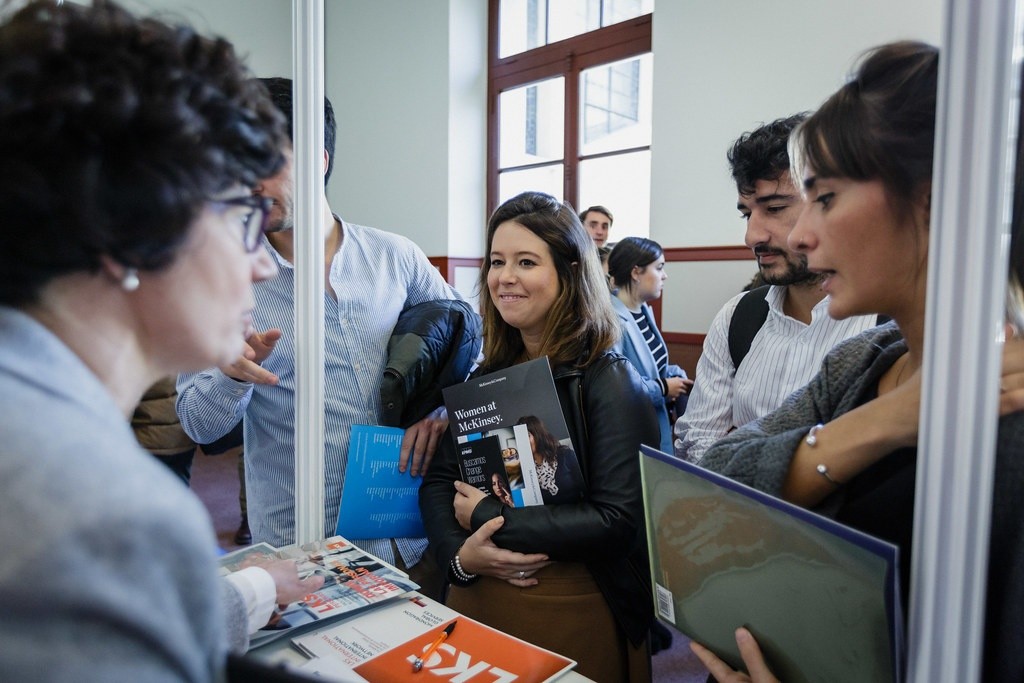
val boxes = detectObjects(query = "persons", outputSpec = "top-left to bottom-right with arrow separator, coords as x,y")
515,416 -> 583,506
492,472 -> 515,508
0,1 -> 696,683
686,40 -> 1024,683
671,107 -> 904,466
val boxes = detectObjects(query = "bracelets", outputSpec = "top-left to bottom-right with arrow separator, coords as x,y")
455,553 -> 480,578
450,559 -> 468,581
804,422 -> 842,491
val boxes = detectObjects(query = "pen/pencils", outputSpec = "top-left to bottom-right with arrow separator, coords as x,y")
411,617 -> 458,669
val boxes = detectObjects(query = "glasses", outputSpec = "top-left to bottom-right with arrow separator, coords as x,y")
202,194 -> 269,254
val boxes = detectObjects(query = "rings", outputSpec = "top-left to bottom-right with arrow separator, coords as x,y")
519,571 -> 526,579
